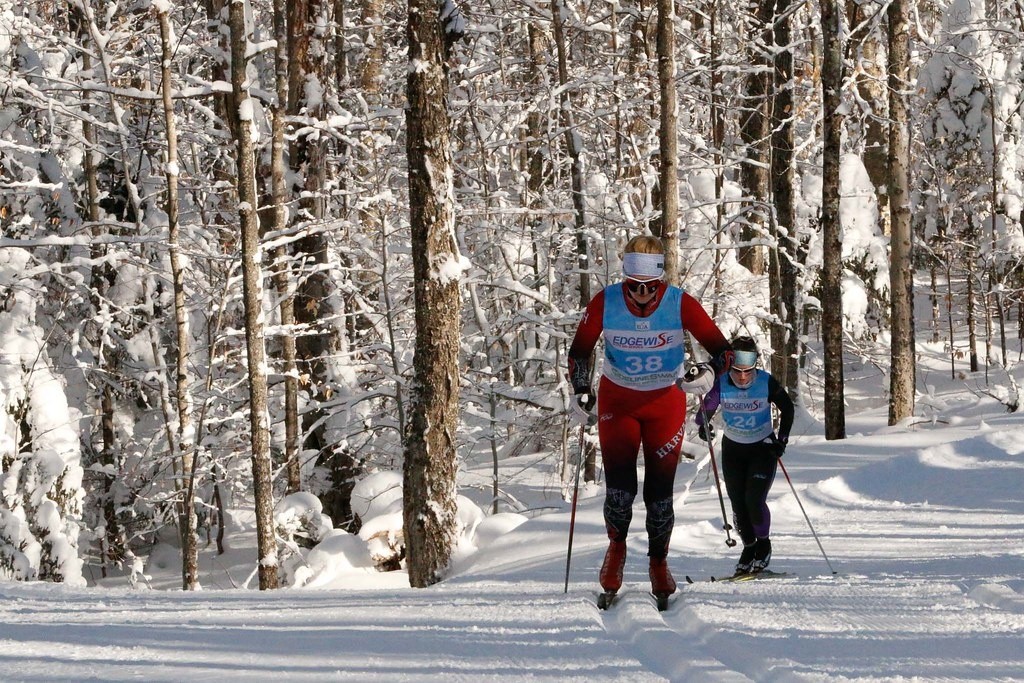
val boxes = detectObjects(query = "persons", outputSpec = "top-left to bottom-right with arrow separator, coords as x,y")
695,338 -> 794,574
568,235 -> 736,597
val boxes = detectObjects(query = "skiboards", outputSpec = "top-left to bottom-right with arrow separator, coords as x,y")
684,568 -> 797,585
595,589 -> 669,612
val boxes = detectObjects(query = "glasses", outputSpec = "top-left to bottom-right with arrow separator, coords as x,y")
623,270 -> 665,295
731,361 -> 757,375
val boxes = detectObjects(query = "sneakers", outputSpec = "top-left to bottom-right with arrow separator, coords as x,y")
649,558 -> 676,595
752,539 -> 771,569
737,541 -> 757,571
600,537 -> 626,592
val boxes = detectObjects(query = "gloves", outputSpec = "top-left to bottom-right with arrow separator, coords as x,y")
572,393 -> 598,426
699,423 -> 715,441
771,439 -> 787,457
678,362 -> 715,395
